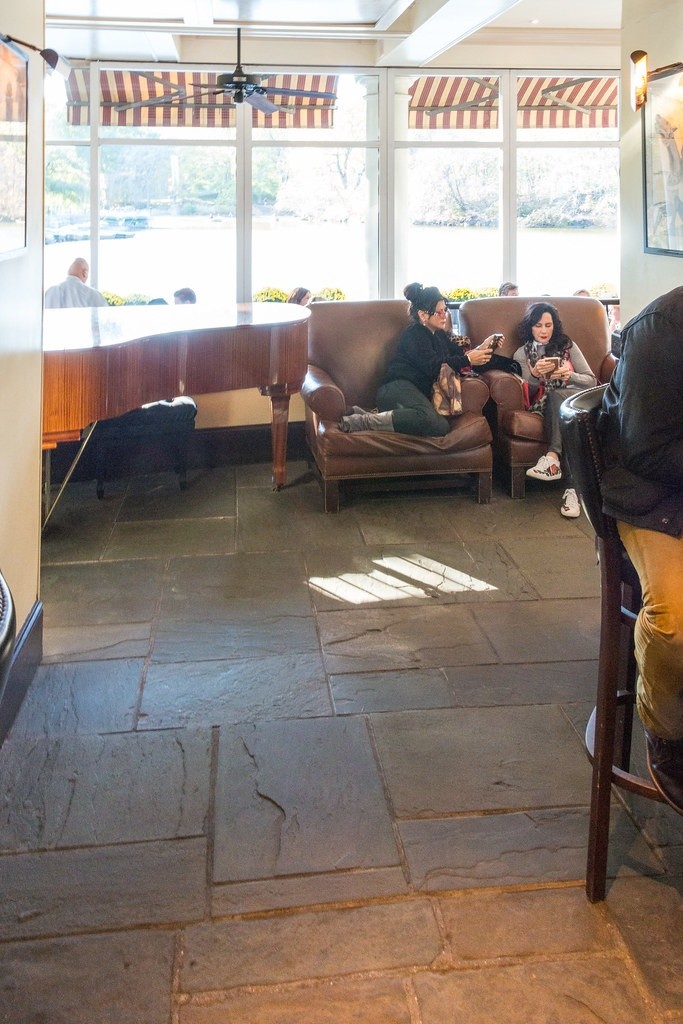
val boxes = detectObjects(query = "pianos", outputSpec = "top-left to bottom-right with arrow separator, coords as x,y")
44,301 -> 314,495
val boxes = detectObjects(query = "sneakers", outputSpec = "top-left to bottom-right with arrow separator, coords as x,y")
561,488 -> 580,517
526,456 -> 562,480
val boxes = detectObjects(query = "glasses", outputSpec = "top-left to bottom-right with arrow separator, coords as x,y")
423,309 -> 448,318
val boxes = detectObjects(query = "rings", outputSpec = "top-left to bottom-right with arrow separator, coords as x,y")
485,361 -> 486,364
561,373 -> 564,377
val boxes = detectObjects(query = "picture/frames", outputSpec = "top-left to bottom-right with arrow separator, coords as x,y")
641,66 -> 683,258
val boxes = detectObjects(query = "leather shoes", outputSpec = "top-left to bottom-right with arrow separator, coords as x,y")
644,728 -> 683,815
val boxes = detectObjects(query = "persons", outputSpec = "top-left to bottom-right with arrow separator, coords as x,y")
498,281 -> 518,296
573,287 -> 589,297
44,257 -> 109,309
608,296 -> 620,331
595,285 -> 683,817
286,287 -> 327,306
339,282 -> 505,436
512,302 -> 597,518
147,287 -> 196,305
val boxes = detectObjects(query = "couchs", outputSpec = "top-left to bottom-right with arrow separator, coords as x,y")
457,296 -> 618,498
301,299 -> 492,513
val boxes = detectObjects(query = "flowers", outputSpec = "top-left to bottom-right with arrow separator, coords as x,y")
254,287 -> 286,303
445,289 -> 497,302
100,291 -> 150,306
311,288 -> 346,300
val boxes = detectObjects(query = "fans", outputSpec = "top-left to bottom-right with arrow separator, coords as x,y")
169,28 -> 337,114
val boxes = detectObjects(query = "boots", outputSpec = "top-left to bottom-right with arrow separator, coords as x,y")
351,406 -> 378,415
342,410 -> 395,433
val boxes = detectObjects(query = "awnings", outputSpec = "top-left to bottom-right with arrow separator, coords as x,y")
0,42 -> 29,121
65,68 -> 339,129
407,75 -> 617,128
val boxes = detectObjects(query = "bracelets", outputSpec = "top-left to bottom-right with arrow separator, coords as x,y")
466,354 -> 471,364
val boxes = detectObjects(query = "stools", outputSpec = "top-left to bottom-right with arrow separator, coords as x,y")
557,383 -> 668,903
81,396 -> 197,498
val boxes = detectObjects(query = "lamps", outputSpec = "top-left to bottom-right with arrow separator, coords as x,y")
4,33 -> 72,79
628,48 -> 683,112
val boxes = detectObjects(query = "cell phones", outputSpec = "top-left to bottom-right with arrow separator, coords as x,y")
545,357 -> 559,379
485,334 -> 503,355
